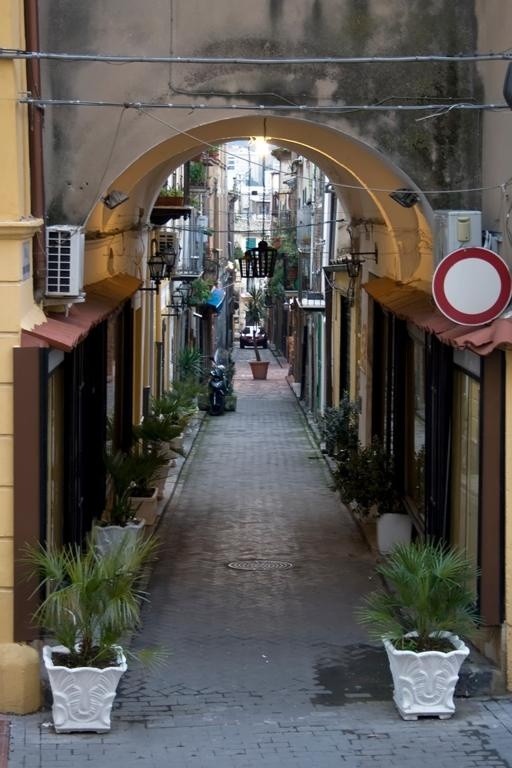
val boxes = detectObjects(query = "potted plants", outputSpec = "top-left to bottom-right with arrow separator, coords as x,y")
23,533 -> 165,734
85,378 -> 209,570
359,540 -> 486,724
334,450 -> 415,556
247,286 -> 270,380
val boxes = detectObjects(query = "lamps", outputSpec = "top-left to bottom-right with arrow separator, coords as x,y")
347,251 -> 382,278
160,283 -> 193,318
101,192 -> 128,210
139,246 -> 178,294
389,188 -> 417,210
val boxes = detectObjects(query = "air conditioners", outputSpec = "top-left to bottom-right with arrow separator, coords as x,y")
434,207 -> 484,271
44,223 -> 86,299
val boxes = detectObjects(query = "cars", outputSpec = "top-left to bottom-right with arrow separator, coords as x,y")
239,326 -> 268,349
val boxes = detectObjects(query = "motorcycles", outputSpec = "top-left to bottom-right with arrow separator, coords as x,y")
208,347 -> 234,416
